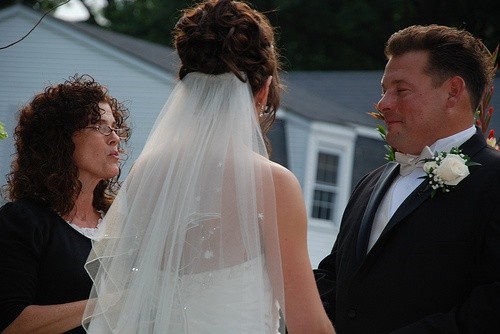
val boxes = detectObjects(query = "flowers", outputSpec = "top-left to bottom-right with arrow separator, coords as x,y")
416,147 -> 470,195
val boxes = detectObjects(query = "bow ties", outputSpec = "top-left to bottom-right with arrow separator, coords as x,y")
394,146 -> 435,177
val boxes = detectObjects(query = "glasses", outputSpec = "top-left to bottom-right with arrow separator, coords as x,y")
81,123 -> 130,140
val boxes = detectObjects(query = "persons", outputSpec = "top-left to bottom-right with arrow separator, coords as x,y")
313,25 -> 500,334
0,73 -> 132,334
126,1 -> 336,334
